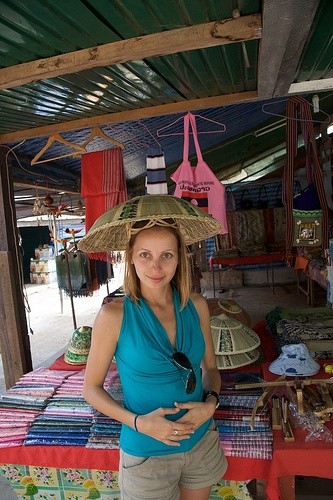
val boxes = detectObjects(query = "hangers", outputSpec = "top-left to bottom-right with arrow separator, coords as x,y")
262,98 -> 331,124
156,111 -> 227,138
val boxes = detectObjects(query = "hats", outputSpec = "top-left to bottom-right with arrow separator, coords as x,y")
200,297 -> 261,370
64,325 -> 92,366
77,193 -> 222,254
268,343 -> 321,377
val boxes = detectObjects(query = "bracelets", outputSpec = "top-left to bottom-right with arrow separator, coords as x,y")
134,416 -> 138,432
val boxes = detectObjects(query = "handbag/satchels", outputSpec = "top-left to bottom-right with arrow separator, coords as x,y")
289,184 -> 325,249
170,112 -> 230,236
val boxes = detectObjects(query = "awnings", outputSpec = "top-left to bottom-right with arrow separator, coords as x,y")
16,215 -> 85,228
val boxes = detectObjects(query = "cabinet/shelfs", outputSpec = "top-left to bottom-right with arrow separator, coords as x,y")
30,133 -> 87,166
73,127 -> 125,159
108,121 -> 164,154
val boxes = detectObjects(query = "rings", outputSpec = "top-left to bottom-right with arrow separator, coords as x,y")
174,430 -> 178,435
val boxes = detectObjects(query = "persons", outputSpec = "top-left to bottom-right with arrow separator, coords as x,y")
76,194 -> 228,500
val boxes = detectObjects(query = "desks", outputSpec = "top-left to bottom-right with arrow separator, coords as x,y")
207,250 -> 296,299
251,319 -> 333,500
0,444 -> 271,500
294,255 -> 328,307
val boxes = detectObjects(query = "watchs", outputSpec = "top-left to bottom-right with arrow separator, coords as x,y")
206,391 -> 220,409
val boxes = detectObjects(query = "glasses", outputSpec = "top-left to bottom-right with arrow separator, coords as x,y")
171,352 -> 197,395
